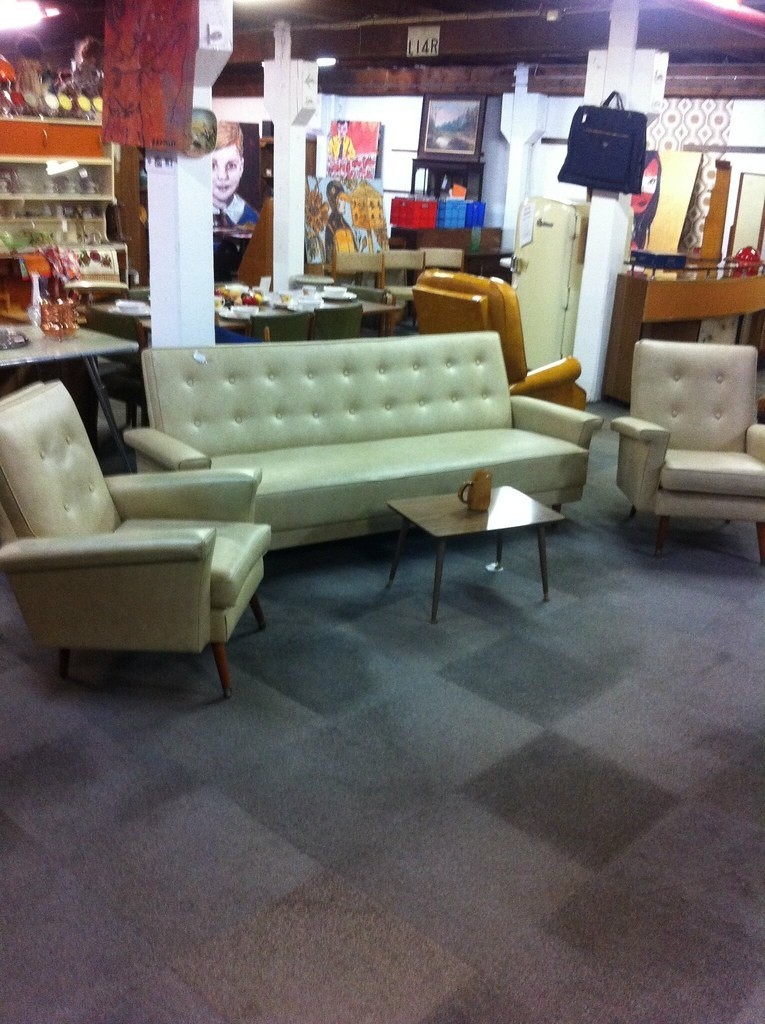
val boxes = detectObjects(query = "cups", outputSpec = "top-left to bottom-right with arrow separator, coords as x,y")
458,470 -> 492,511
215,297 -> 225,311
303,286 -> 316,298
279,292 -> 293,303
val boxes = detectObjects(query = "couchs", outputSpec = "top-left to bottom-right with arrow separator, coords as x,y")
412,266 -> 587,414
0,379 -> 270,702
609,338 -> 764,567
121,330 -> 604,552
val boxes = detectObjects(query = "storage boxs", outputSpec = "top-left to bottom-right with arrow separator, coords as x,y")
390,195 -> 486,230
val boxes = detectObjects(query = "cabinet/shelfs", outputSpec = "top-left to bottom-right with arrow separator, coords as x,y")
0,115 -> 128,290
410,159 -> 485,203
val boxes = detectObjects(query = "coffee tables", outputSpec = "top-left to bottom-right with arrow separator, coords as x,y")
385,484 -> 565,623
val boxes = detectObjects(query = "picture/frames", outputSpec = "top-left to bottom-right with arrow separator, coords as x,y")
415,91 -> 488,163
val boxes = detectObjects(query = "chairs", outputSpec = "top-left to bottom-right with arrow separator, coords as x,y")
378,249 -> 427,300
84,282 -> 396,430
419,246 -> 465,272
330,252 -> 384,288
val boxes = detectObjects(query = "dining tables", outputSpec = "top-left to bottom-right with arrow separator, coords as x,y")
75,289 -> 402,431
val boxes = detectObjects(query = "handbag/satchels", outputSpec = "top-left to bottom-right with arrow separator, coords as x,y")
557,92 -> 647,195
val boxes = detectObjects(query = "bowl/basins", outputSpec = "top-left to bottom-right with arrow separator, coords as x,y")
297,300 -> 323,306
325,287 -> 346,296
233,307 -> 258,317
117,302 -> 145,312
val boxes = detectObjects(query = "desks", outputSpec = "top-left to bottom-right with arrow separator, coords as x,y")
600,267 -> 764,403
0,322 -> 139,476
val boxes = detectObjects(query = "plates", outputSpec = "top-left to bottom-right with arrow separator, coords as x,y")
110,308 -> 151,316
219,312 -> 274,319
321,293 -> 357,299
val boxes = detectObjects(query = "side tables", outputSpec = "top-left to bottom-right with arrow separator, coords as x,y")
464,247 -> 514,277
